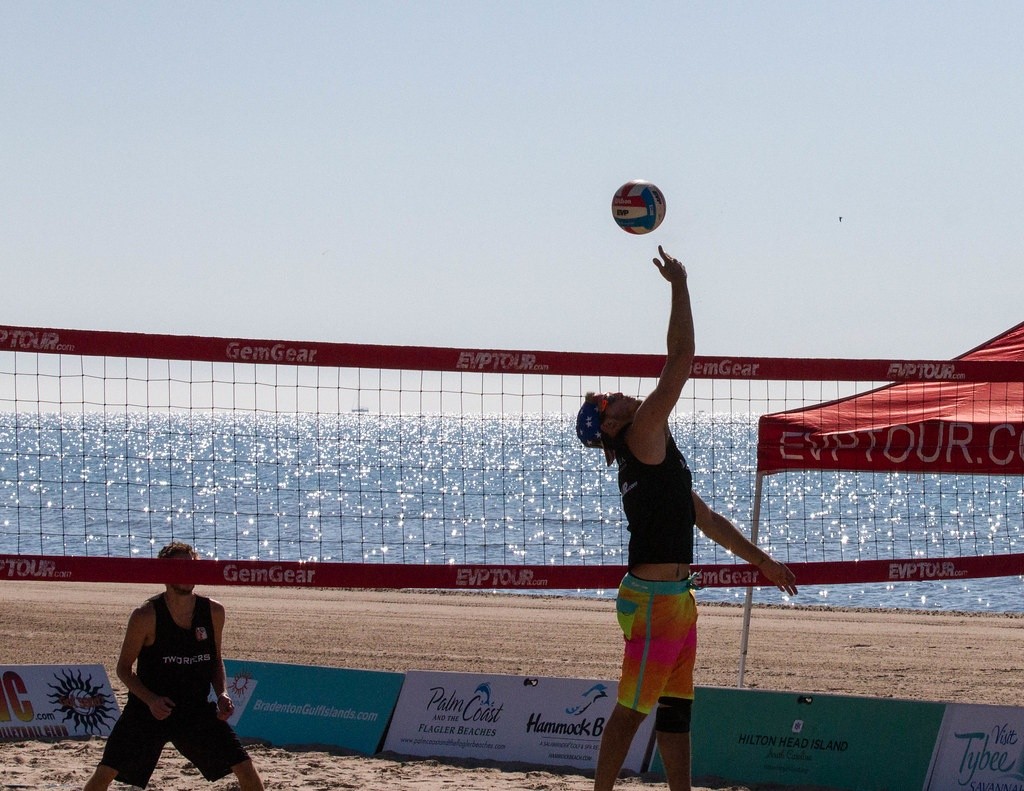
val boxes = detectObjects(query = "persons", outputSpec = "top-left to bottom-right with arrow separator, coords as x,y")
574,245 -> 798,791
83,542 -> 266,791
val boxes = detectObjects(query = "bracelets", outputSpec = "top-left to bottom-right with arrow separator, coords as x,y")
758,554 -> 769,568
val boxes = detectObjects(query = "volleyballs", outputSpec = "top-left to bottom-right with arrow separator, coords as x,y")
611,179 -> 666,235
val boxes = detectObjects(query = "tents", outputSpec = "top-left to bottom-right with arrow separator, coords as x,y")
737,321 -> 1024,693
